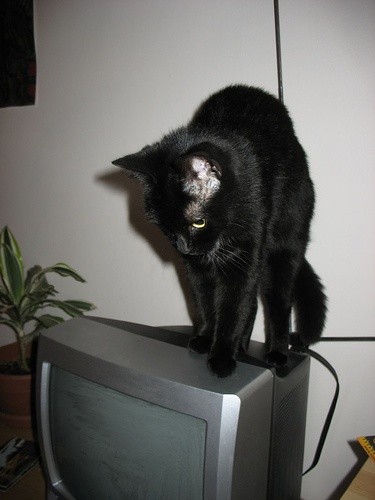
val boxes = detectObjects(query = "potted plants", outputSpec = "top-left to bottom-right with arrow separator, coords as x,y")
0,225 -> 98,442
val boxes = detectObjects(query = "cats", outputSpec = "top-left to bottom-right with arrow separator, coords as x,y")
112,85 -> 326,379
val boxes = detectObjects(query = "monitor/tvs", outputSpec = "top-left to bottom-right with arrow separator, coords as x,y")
35,316 -> 310,500
